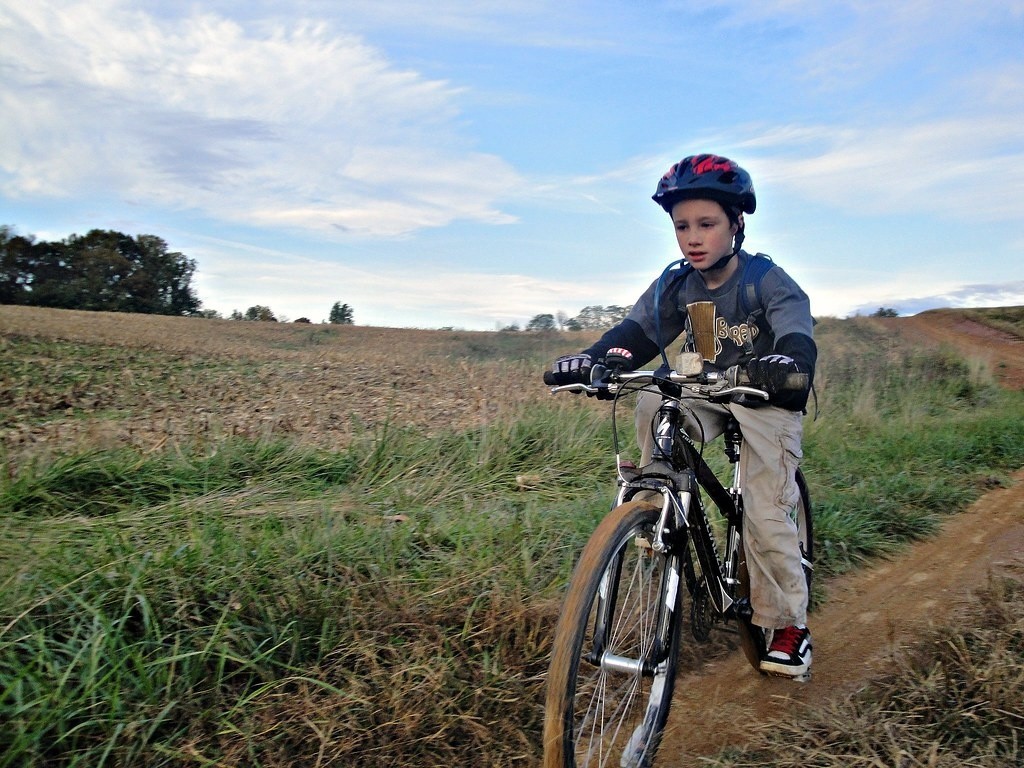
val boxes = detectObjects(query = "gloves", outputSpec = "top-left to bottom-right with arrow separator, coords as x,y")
551,353 -> 595,397
746,354 -> 800,394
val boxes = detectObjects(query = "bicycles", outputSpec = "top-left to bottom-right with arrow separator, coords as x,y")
544,348 -> 816,768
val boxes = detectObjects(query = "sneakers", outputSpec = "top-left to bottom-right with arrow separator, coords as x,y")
759,624 -> 813,676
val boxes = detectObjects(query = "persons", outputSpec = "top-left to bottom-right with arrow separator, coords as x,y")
552,153 -> 816,676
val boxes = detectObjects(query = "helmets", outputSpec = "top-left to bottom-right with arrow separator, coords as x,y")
652,154 -> 756,214
